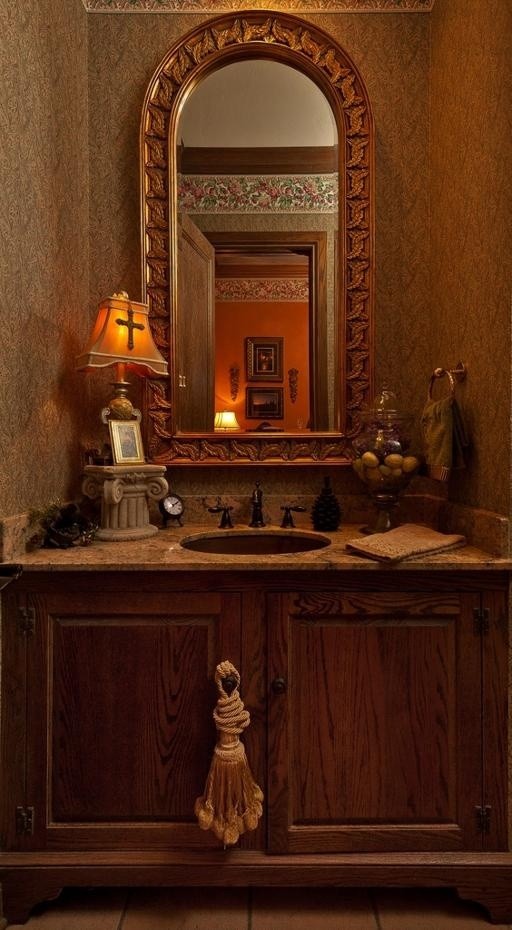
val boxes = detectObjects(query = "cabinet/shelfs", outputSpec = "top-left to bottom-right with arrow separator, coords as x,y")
24,590 -> 484,854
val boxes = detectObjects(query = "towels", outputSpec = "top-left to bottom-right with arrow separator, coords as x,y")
346,523 -> 466,564
419,393 -> 468,481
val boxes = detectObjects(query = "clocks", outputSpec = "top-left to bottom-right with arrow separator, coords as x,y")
158,494 -> 185,528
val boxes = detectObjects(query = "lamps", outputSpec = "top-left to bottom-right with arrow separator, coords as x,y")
214,409 -> 241,431
72,290 -> 170,419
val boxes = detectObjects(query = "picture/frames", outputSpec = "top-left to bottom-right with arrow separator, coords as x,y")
108,419 -> 146,466
245,386 -> 284,420
245,337 -> 284,382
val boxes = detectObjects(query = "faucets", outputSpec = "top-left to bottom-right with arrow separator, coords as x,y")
247,481 -> 267,527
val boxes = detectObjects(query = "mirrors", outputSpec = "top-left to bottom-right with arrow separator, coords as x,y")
136,8 -> 377,466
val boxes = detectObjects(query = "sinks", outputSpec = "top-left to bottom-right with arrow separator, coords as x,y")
178,528 -> 332,556
